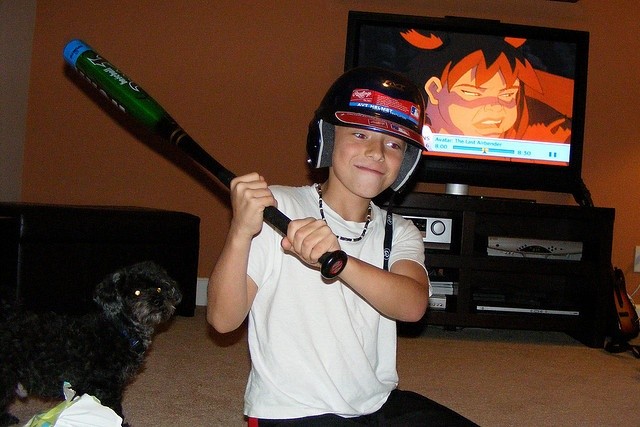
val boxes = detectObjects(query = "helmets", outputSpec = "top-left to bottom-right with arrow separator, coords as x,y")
308,66 -> 428,191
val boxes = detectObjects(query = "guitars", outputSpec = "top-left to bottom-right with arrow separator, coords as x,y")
571,178 -> 640,344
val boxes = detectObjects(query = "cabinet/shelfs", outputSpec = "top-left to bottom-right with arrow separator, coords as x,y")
371,193 -> 615,349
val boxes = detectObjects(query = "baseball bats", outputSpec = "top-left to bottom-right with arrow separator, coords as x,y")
63,40 -> 348,280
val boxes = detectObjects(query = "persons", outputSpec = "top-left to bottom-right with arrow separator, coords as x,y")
206,67 -> 484,426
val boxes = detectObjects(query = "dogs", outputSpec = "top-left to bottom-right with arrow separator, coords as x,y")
0,260 -> 182,426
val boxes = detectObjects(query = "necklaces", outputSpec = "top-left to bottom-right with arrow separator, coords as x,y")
318,183 -> 372,242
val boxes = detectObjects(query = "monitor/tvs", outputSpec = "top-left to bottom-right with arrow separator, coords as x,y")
344,9 -> 591,204
